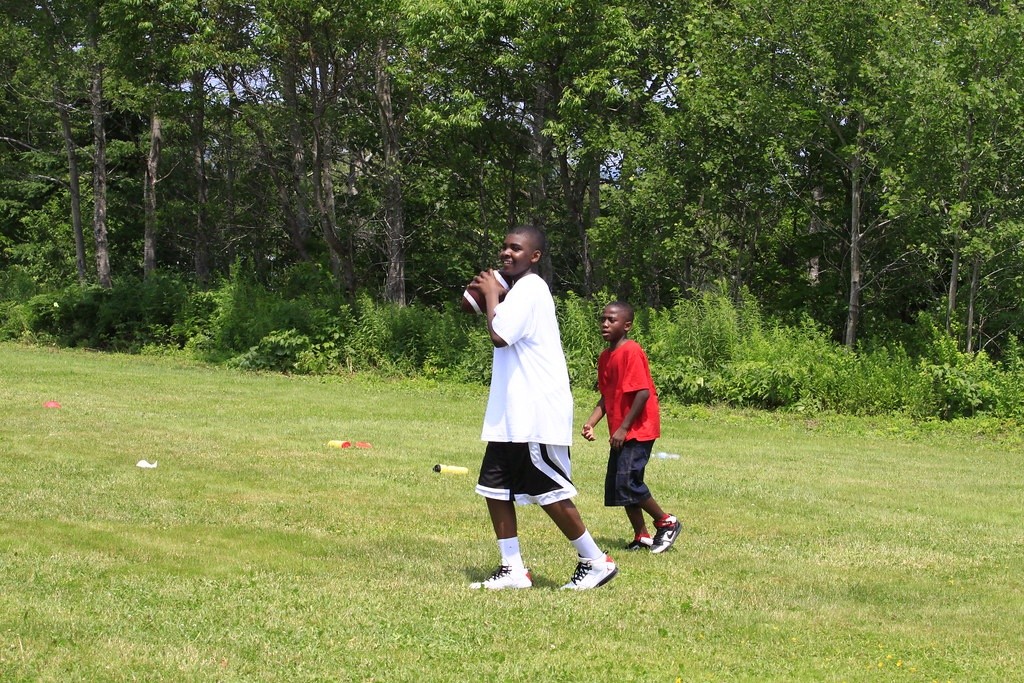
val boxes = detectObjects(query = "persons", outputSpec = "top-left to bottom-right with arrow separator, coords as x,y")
581,300 -> 682,554
467,225 -> 618,591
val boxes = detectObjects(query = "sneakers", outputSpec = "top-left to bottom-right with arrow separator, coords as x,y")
649,513 -> 682,554
624,534 -> 654,550
469,565 -> 532,590
559,550 -> 618,591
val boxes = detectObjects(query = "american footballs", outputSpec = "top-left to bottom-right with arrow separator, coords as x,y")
461,268 -> 517,315
